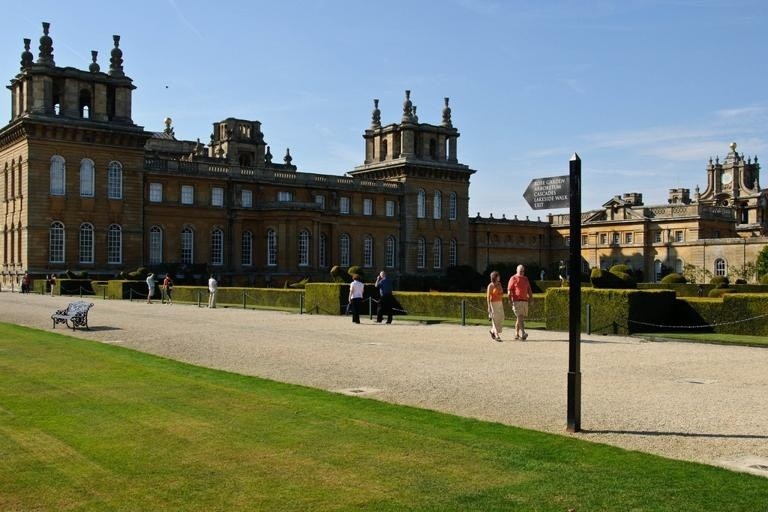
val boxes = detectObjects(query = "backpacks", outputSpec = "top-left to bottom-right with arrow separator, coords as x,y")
168,279 -> 172,291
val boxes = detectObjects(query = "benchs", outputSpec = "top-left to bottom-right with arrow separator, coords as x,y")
51,302 -> 94,332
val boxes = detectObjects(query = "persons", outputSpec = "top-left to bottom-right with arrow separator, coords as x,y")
21,272 -> 31,293
49,276 -> 56,297
146,273 -> 156,304
506,265 -> 534,341
162,273 -> 173,306
486,270 -> 506,342
207,273 -> 218,309
374,270 -> 395,325
348,273 -> 365,323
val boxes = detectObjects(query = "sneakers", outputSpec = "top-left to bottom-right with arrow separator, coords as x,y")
515,332 -> 528,341
489,330 -> 501,341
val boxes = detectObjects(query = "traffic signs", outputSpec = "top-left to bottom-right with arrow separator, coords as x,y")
522,175 -> 570,210
